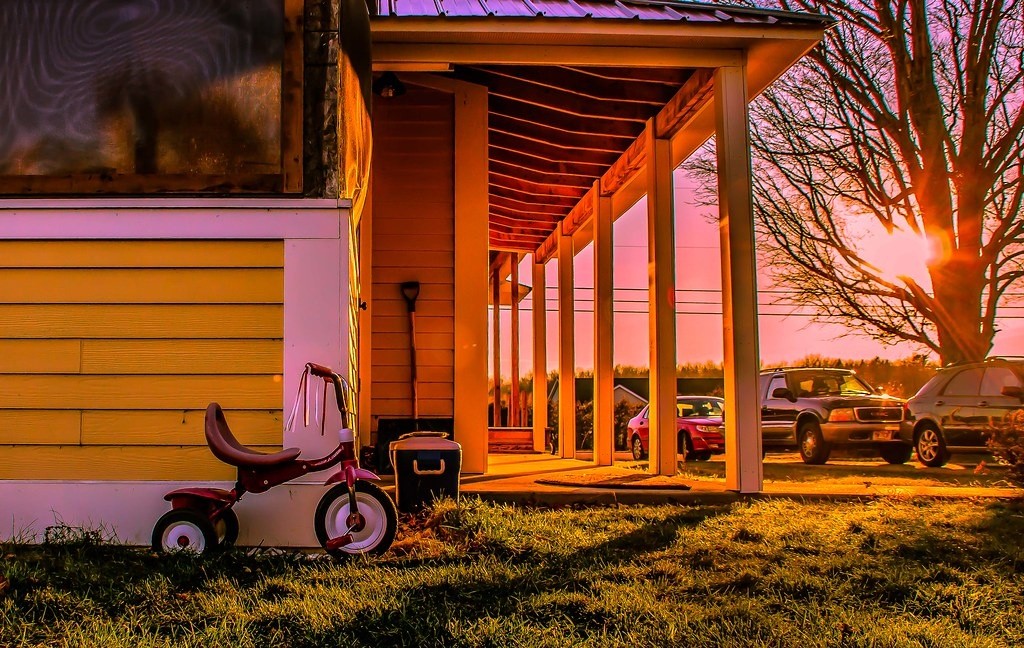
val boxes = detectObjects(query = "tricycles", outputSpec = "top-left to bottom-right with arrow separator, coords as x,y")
151,362 -> 399,583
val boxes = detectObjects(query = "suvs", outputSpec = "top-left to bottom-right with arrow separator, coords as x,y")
758,366 -> 912,466
899,356 -> 1024,469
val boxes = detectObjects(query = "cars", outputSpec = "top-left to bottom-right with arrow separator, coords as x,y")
623,395 -> 727,461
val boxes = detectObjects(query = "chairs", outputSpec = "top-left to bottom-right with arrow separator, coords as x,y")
682,409 -> 692,417
812,378 -> 831,395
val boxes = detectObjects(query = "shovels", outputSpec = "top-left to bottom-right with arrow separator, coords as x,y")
373,281 -> 453,477
